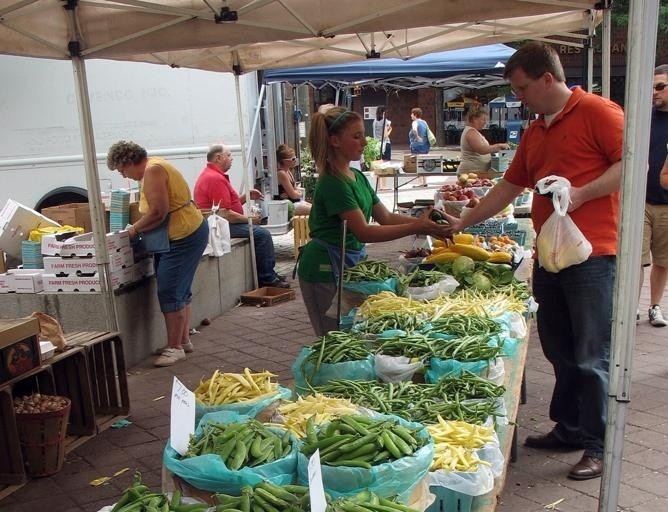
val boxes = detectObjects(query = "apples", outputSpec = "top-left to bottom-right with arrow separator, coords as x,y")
442,191 -> 475,202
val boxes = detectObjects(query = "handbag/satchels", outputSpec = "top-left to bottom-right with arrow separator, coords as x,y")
420,119 -> 437,145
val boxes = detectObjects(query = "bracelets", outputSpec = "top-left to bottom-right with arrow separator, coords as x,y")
132,224 -> 139,235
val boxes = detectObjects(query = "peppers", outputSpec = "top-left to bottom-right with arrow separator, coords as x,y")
400,267 -> 448,288
306,329 -> 375,374
426,305 -> 507,336
375,336 -> 507,366
340,254 -> 399,284
296,367 -> 507,424
359,310 -> 426,334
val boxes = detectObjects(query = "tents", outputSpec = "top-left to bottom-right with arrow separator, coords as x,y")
1,1 -> 659,512
264,42 -> 518,104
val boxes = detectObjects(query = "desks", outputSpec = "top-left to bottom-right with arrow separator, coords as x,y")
380,172 -> 458,213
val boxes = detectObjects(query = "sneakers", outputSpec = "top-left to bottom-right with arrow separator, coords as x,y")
636,309 -> 641,325
155,347 -> 186,368
648,305 -> 668,326
158,340 -> 194,355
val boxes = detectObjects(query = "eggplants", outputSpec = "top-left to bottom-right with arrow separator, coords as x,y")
453,256 -> 475,278
481,261 -> 513,273
470,271 -> 491,293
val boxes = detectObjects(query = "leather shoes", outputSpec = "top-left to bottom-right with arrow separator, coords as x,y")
526,426 -> 581,453
568,452 -> 615,479
277,276 -> 288,281
269,281 -> 289,289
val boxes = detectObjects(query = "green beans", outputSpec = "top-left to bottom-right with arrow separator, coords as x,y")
329,488 -> 419,512
355,283 -> 528,323
426,414 -> 497,473
193,367 -> 279,406
212,481 -> 329,512
111,470 -> 211,512
276,393 -> 359,440
300,413 -> 425,470
183,420 -> 291,471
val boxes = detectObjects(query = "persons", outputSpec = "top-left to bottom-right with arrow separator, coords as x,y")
409,108 -> 437,188
294,106 -> 453,338
106,140 -> 209,368
372,105 -> 393,162
660,142 -> 667,191
193,144 -> 291,290
635,64 -> 668,327
432,42 -> 624,481
457,103 -> 511,181
271,143 -> 312,217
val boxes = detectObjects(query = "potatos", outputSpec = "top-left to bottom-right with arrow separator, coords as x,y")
459,172 -> 478,184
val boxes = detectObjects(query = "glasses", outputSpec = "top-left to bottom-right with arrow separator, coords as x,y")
511,71 -> 553,96
282,157 -> 298,162
654,85 -> 668,92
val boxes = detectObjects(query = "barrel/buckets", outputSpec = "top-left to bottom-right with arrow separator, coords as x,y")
13,393 -> 72,478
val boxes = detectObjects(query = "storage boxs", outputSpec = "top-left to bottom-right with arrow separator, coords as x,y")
416,154 -> 444,174
41,203 -> 106,233
403,155 -> 417,172
0,316 -> 41,385
